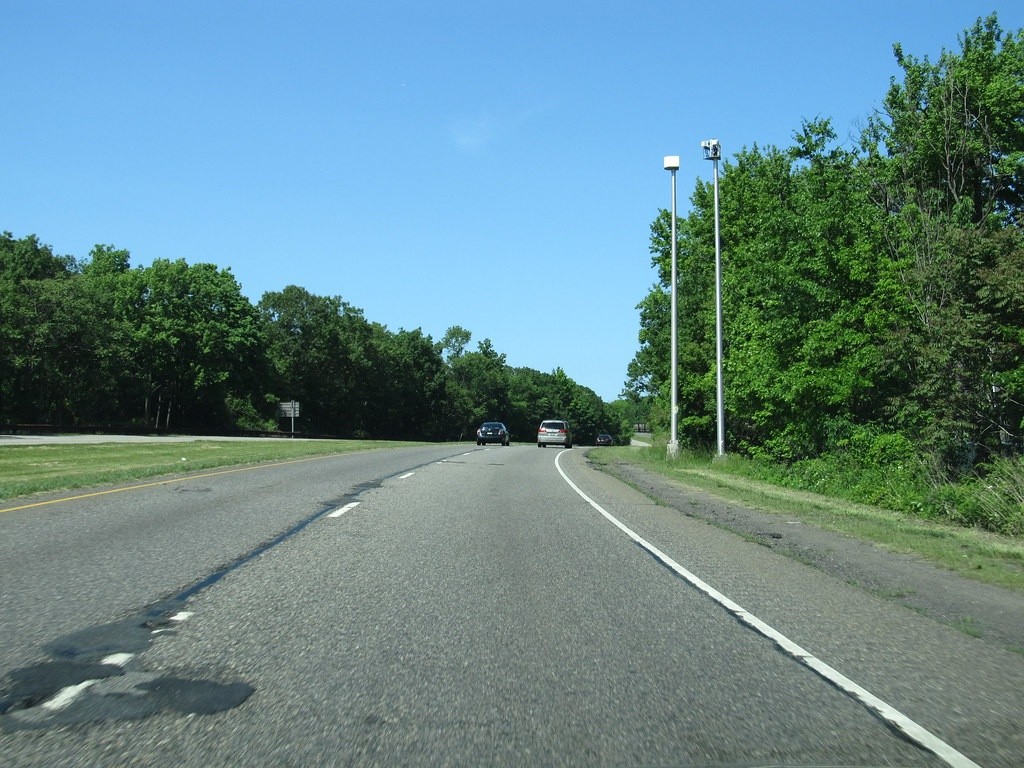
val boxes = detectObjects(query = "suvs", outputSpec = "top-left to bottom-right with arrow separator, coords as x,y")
537,420 -> 573,448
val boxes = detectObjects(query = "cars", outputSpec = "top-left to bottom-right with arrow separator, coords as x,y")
476,422 -> 511,446
595,435 -> 614,446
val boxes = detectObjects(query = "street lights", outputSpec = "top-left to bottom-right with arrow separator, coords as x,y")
703,140 -> 721,454
663,155 -> 682,445
